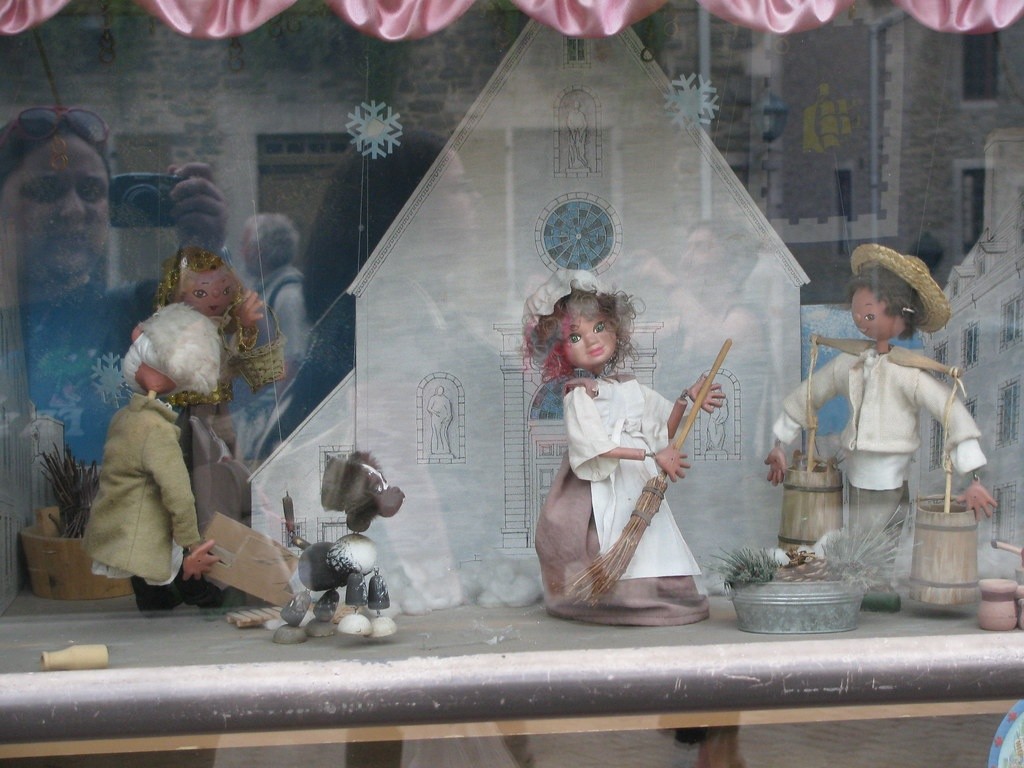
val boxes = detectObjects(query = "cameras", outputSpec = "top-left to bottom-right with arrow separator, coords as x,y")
108,174 -> 188,228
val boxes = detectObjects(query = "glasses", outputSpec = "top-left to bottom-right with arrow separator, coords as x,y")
0,104 -> 110,156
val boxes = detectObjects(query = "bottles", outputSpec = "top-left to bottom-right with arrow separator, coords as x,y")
38,643 -> 109,673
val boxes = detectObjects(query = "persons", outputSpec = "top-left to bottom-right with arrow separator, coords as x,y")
239,213 -> 306,396
0,104 -> 230,467
83,302 -> 225,620
153,246 -> 265,538
764,240 -> 1001,570
524,265 -> 728,581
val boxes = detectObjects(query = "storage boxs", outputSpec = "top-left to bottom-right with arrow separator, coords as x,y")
199,511 -> 302,611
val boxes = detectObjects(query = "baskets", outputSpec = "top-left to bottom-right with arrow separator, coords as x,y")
220,297 -> 288,394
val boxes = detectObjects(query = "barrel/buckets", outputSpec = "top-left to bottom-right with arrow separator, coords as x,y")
909,494 -> 984,610
777,464 -> 844,559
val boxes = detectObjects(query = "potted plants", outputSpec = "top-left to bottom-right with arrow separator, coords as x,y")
696,503 -> 927,638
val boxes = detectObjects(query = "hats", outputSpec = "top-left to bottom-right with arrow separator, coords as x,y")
850,243 -> 952,333
